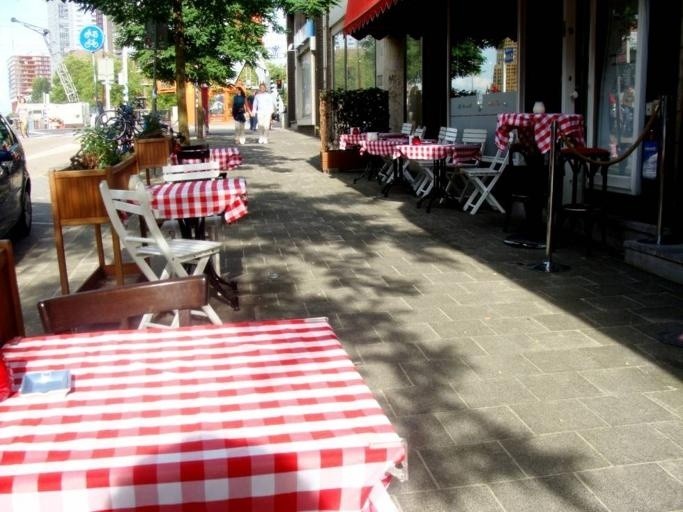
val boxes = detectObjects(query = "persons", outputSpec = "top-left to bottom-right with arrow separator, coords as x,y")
251,83 -> 275,145
232,86 -> 251,146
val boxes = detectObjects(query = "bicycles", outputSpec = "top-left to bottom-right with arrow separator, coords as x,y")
95,95 -> 173,154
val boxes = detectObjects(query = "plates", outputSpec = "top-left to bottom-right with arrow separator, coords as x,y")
18,369 -> 71,399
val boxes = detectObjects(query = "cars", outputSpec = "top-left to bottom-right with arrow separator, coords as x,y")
1,112 -> 33,238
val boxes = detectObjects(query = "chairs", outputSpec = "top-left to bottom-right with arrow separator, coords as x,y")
36,275 -> 210,336
349,122 -> 514,215
176,143 -> 210,164
550,150 -> 625,262
98,179 -> 224,330
161,160 -> 223,298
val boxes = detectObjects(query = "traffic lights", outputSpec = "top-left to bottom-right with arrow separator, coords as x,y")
277,79 -> 282,89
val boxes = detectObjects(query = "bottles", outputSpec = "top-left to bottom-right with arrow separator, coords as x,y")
533,101 -> 546,114
353,127 -> 358,135
412,135 -> 421,146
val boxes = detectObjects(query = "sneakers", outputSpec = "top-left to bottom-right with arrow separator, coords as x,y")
258,136 -> 268,144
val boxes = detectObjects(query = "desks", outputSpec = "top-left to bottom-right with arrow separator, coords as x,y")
0,316 -> 411,512
339,133 -> 381,184
167,147 -> 243,173
134,175 -> 250,311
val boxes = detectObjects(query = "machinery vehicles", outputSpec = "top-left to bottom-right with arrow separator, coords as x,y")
10,17 -> 91,129
